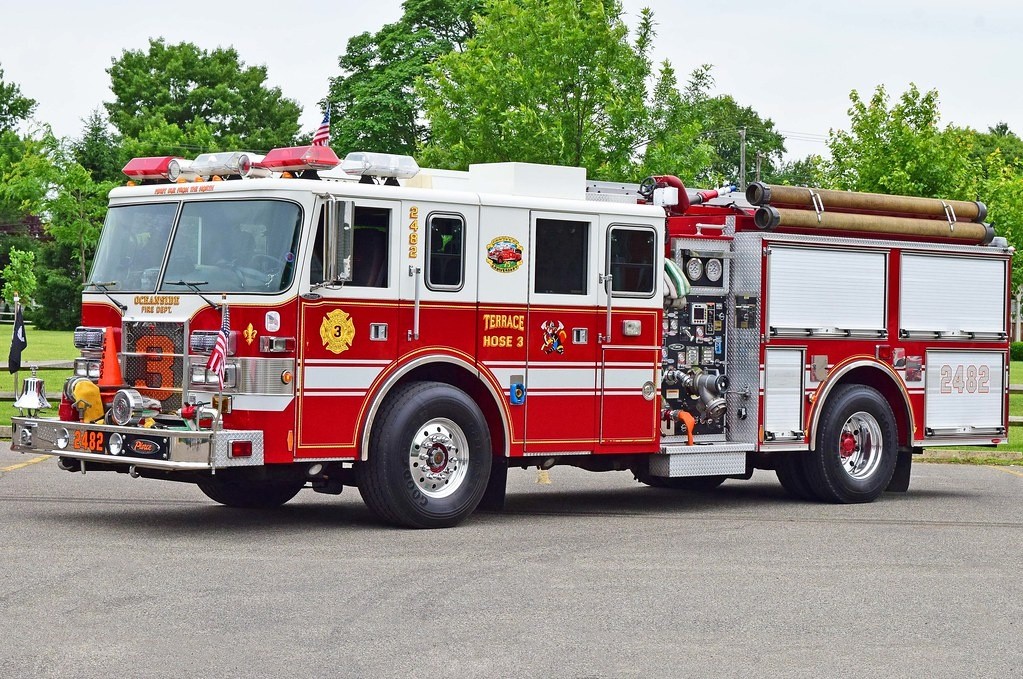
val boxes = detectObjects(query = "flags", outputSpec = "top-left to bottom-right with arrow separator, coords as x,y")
9,305 -> 28,374
206,303 -> 230,391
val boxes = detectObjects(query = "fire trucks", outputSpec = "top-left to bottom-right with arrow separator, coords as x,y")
10,137 -> 1023,532
